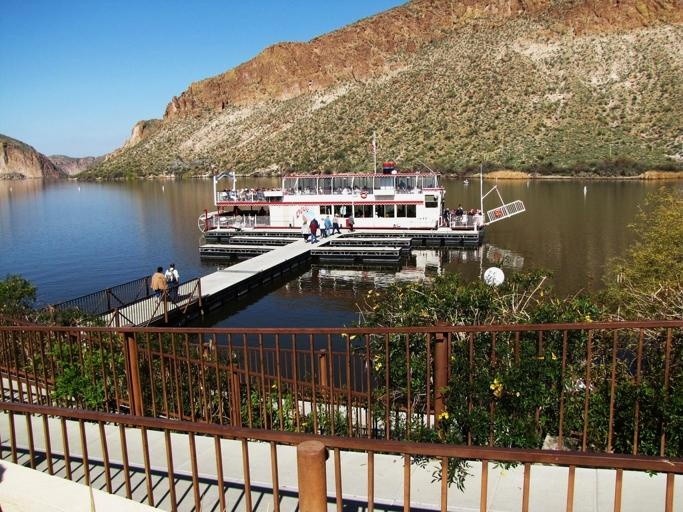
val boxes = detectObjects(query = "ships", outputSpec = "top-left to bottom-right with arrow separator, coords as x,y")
197,129 -> 525,233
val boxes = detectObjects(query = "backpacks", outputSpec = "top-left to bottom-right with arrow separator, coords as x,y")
165,271 -> 175,284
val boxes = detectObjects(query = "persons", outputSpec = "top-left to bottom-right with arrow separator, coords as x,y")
219,179 -> 483,244
164,264 -> 179,304
150,267 -> 168,304
